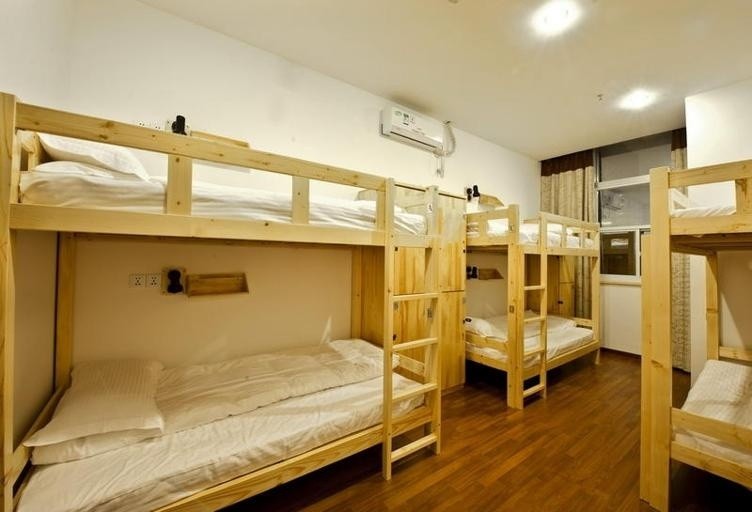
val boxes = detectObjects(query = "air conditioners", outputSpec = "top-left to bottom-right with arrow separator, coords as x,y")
378,107 -> 456,179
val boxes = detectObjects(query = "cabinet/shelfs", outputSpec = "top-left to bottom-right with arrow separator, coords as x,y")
361,180 -> 465,395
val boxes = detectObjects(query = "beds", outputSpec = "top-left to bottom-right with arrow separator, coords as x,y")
466,204 -> 601,409
0,92 -> 442,511
639,158 -> 752,512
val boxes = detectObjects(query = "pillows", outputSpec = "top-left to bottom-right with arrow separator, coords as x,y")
24,356 -> 160,447
39,131 -> 146,179
466,315 -> 505,339
